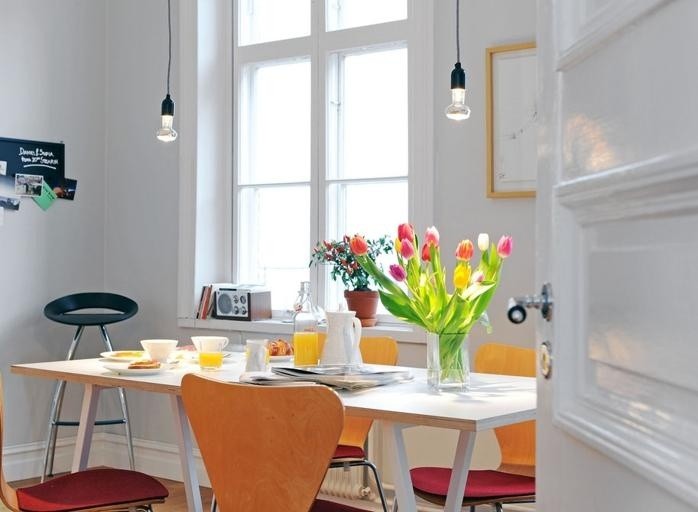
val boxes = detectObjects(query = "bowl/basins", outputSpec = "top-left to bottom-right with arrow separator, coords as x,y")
140,338 -> 178,362
189,336 -> 229,355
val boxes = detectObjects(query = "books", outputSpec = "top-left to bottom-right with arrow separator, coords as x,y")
196,285 -> 208,318
241,362 -> 418,392
202,282 -> 239,319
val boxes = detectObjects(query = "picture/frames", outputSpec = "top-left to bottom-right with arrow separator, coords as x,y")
484,40 -> 537,199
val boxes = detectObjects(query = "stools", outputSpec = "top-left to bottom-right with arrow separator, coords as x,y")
38,292 -> 138,485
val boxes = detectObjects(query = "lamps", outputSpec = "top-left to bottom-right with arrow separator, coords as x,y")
155,1 -> 179,144
445,0 -> 471,122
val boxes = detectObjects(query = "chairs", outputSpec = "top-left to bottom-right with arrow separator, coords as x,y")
406,343 -> 537,512
0,378 -> 167,512
181,373 -> 366,512
207,334 -> 398,512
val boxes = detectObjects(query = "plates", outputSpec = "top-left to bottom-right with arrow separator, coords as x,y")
99,350 -> 146,361
105,364 -> 169,376
269,354 -> 293,363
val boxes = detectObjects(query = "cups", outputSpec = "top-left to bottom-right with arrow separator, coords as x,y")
244,338 -> 272,374
292,282 -> 318,366
200,341 -> 224,371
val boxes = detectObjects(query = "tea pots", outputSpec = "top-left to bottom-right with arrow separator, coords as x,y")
323,304 -> 365,363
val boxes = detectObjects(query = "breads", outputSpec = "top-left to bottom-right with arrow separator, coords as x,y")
267,339 -> 293,356
128,360 -> 162,370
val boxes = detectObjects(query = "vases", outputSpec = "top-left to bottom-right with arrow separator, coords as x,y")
425,332 -> 468,392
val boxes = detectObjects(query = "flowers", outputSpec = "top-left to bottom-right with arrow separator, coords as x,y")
309,237 -> 397,290
350,224 -> 511,378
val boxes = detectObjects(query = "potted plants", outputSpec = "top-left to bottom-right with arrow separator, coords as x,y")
342,288 -> 379,328
